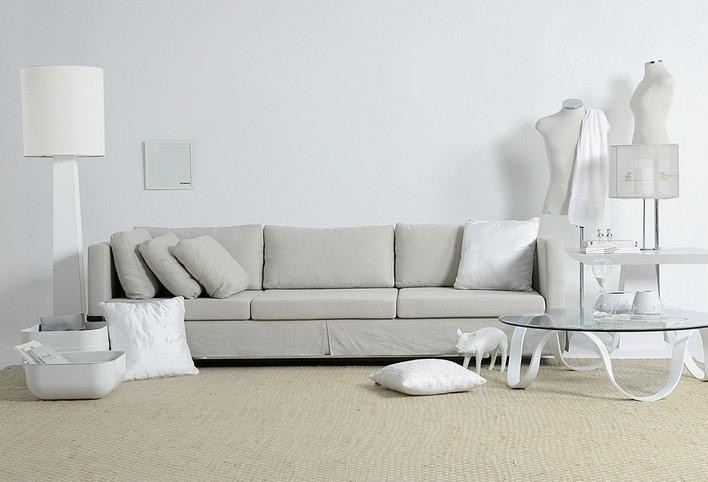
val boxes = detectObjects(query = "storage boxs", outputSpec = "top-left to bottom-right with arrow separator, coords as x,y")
25,346 -> 126,401
21,320 -> 111,351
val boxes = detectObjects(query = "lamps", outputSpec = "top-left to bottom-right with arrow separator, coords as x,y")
17,65 -> 108,320
607,142 -> 681,251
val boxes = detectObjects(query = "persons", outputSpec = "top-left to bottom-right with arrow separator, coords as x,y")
630,61 -> 678,146
536,97 -> 612,217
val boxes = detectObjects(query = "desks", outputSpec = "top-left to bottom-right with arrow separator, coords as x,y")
563,239 -> 708,359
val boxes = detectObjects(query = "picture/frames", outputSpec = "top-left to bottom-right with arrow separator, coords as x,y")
143,139 -> 194,191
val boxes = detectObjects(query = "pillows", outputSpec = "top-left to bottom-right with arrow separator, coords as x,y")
97,295 -> 200,382
39,311 -> 87,331
170,235 -> 250,298
108,225 -> 159,301
137,231 -> 204,300
452,216 -> 540,293
367,358 -> 487,396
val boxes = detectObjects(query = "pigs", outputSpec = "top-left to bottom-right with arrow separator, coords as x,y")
454,326 -> 508,375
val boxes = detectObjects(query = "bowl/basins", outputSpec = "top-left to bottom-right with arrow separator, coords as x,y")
593,291 -> 636,310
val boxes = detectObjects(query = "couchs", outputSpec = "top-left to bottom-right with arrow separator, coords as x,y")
84,216 -> 569,364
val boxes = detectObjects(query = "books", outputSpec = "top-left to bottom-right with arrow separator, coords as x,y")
580,239 -> 641,254
15,340 -> 69,364
